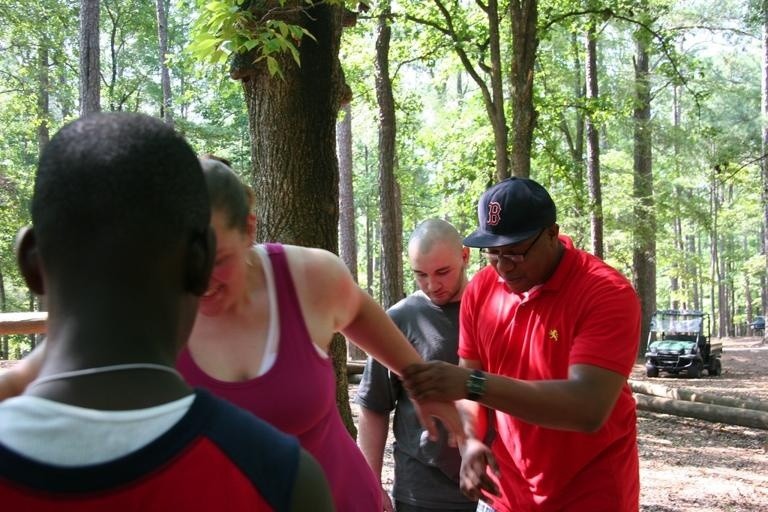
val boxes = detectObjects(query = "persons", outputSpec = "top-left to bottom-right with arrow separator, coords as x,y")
400,176 -> 645,512
0,152 -> 469,512
353,214 -> 478,512
1,108 -> 340,512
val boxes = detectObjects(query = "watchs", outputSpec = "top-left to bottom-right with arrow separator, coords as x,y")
464,369 -> 488,402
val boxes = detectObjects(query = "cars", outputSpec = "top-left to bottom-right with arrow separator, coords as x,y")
751,314 -> 765,330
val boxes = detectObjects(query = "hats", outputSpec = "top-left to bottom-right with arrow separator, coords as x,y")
461,176 -> 557,248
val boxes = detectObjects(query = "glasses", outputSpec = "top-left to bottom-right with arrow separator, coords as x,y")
479,227 -> 547,262
